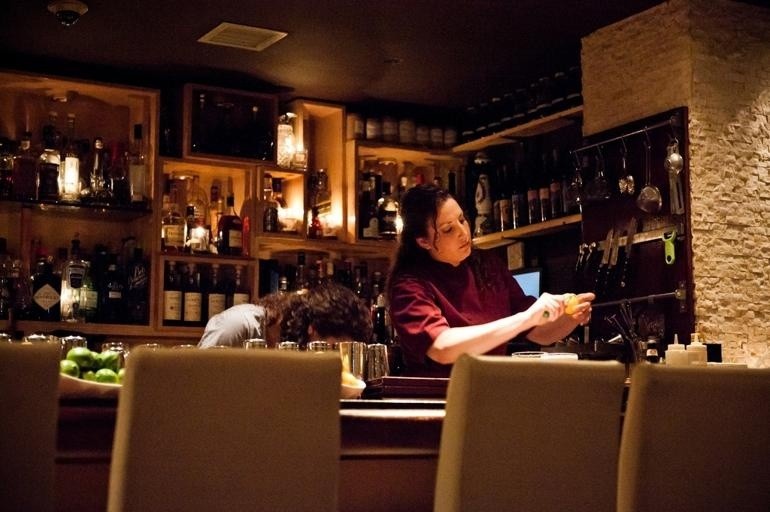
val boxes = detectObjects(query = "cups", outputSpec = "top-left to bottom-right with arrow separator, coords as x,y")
366,344 -> 390,380
333,341 -> 366,384
512,350 -> 549,360
543,352 -> 579,360
289,148 -> 310,172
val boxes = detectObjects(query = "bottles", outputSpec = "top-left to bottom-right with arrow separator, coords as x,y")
358,164 -> 460,238
644,334 -> 659,367
23,334 -> 49,345
142,341 -> 163,352
372,270 -> 386,285
241,336 -> 268,351
161,168 -> 251,327
312,255 -> 327,287
174,343 -> 194,351
192,92 -> 271,159
101,341 -> 130,366
461,64 -> 582,141
686,333 -> 709,370
264,173 -> 294,235
368,283 -> 385,327
306,340 -> 328,353
62,336 -> 88,360
278,278 -> 289,294
276,340 -> 302,353
208,345 -> 231,351
470,146 -> 579,230
352,267 -> 365,295
6,111 -> 151,323
292,251 -> 311,291
346,107 -> 458,151
664,332 -> 689,369
341,259 -> 354,287
326,262 -> 337,282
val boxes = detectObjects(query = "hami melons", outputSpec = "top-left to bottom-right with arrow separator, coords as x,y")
341,371 -> 366,399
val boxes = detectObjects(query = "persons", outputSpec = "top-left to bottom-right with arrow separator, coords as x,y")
382,181 -> 597,375
197,285 -> 373,352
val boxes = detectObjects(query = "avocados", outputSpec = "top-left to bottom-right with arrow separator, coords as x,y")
60,360 -> 81,378
101,350 -> 121,372
95,368 -> 117,384
90,351 -> 104,370
117,368 -> 125,384
66,347 -> 93,369
82,371 -> 95,382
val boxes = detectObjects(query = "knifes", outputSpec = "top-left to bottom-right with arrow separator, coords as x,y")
589,228 -> 615,294
619,216 -> 638,288
602,229 -> 622,295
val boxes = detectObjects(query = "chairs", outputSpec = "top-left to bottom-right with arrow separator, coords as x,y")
0,340 -> 65,510
618,360 -> 769,512
431,351 -> 626,511
109,344 -> 343,512
